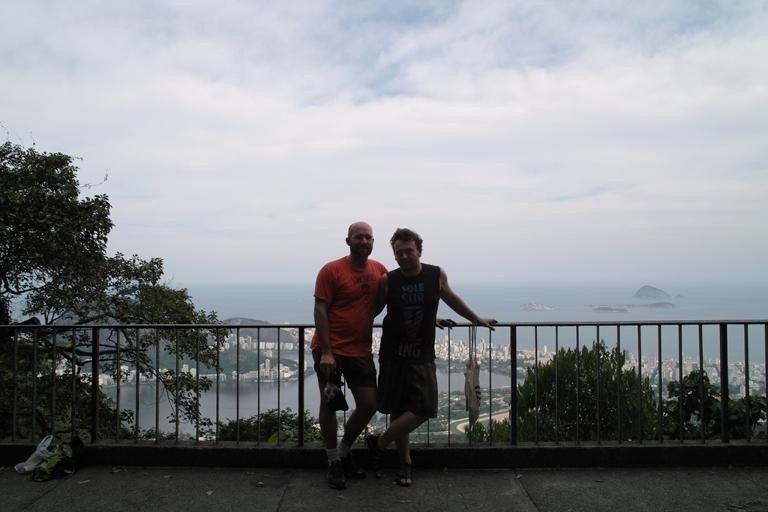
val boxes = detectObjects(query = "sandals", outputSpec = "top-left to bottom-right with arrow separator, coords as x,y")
366,433 -> 387,477
396,455 -> 415,487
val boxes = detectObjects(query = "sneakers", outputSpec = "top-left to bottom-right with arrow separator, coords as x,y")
327,460 -> 348,490
340,451 -> 366,480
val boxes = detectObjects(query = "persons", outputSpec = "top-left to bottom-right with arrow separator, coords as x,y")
310,220 -> 459,491
364,227 -> 498,487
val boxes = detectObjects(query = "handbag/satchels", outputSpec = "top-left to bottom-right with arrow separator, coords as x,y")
464,321 -> 482,425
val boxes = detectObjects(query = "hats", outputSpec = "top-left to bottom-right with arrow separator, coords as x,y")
322,368 -> 348,411
